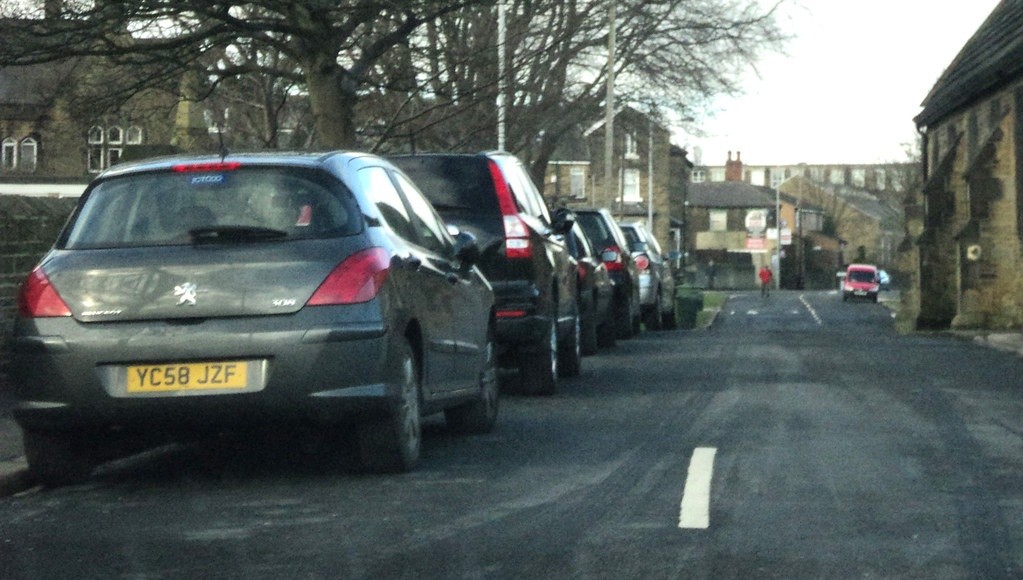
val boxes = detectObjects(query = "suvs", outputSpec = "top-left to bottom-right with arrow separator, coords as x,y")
616,220 -> 676,333
8,150 -> 503,488
574,206 -> 649,340
383,150 -> 583,398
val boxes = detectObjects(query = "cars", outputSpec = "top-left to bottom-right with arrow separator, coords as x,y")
668,252 -> 699,330
549,206 -> 619,354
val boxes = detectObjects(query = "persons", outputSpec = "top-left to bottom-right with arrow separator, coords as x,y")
758,265 -> 774,296
705,257 -> 718,288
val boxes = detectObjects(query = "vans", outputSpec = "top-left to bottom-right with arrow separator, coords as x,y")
842,263 -> 881,303
